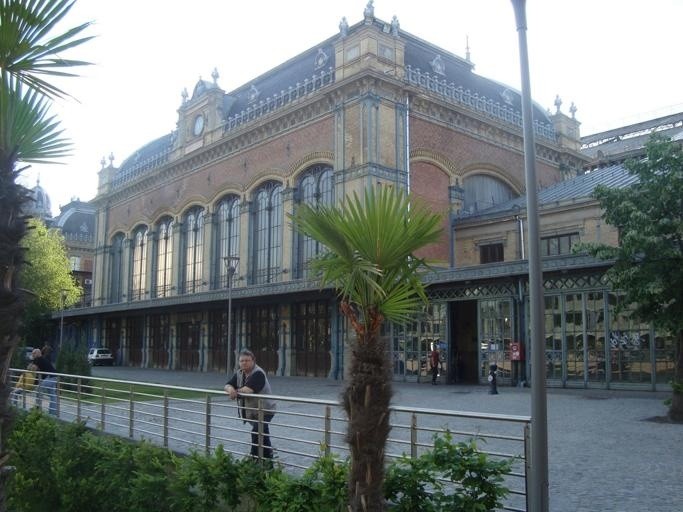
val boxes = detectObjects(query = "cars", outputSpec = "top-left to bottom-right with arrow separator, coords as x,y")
87,347 -> 114,366
18,347 -> 34,360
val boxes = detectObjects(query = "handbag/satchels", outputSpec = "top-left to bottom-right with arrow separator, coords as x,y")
15,364 -> 37,391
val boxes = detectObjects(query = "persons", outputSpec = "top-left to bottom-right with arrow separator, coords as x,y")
31,348 -> 58,416
10,362 -> 36,406
224,349 -> 276,470
430,345 -> 441,384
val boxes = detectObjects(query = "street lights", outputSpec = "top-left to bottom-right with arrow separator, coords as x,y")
224,257 -> 240,376
60,289 -> 70,352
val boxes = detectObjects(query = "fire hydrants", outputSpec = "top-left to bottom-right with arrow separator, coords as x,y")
488,365 -> 498,394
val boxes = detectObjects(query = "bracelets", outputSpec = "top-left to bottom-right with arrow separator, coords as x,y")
237,389 -> 242,392
